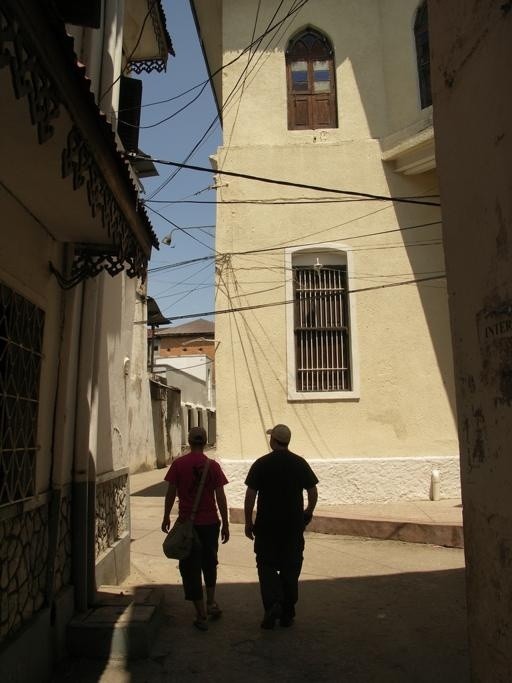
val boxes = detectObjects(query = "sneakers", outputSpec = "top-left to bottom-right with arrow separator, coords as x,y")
192,600 -> 223,631
260,613 -> 295,629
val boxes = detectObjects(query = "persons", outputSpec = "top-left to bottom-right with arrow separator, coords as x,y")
160,425 -> 231,633
243,422 -> 320,631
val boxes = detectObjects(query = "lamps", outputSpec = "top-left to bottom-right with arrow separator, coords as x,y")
160,225 -> 216,247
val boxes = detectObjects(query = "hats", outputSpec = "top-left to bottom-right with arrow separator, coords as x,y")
188,427 -> 207,443
266,424 -> 291,443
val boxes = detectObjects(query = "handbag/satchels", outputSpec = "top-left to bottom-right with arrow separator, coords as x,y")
162,518 -> 202,560
